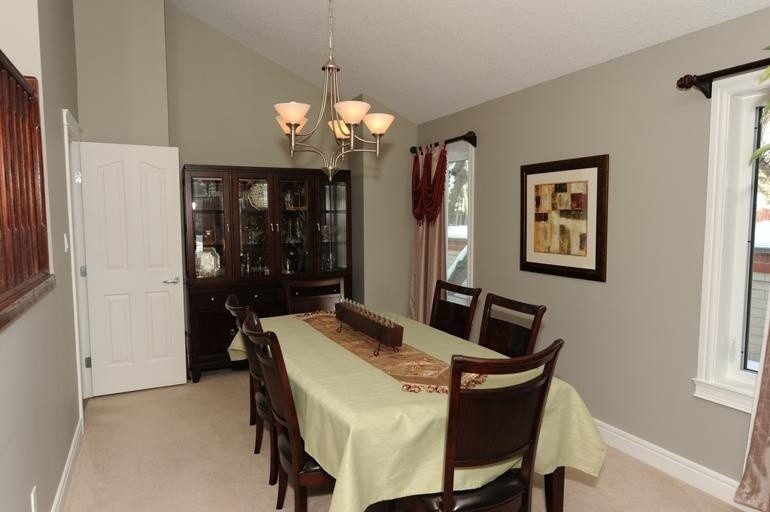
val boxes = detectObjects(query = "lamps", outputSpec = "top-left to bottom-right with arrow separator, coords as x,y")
272,1 -> 394,182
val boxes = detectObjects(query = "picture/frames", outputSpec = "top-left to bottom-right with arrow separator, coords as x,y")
519,154 -> 609,283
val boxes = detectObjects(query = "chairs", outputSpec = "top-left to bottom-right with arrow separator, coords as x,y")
385,338 -> 564,511
224,293 -> 267,453
478,293 -> 547,358
428,279 -> 482,342
285,281 -> 343,315
242,312 -> 337,511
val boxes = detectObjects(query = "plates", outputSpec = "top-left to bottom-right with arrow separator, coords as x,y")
247,179 -> 269,212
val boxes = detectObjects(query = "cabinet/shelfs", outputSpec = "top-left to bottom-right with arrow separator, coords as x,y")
182,163 -> 235,286
186,286 -> 282,384
313,169 -> 352,279
230,165 -> 315,285
